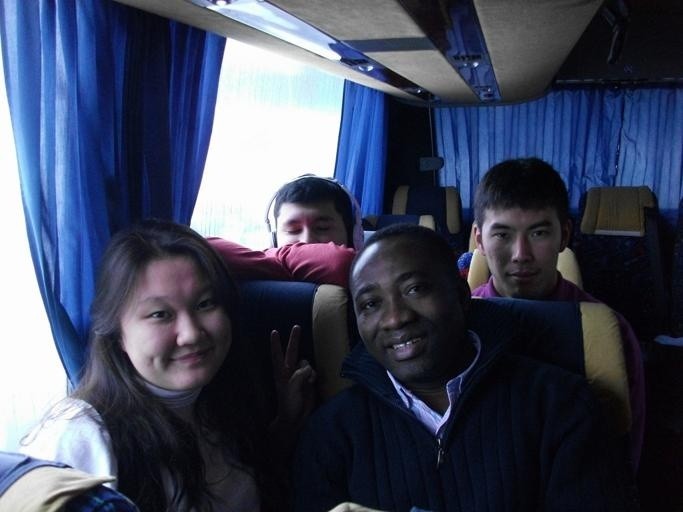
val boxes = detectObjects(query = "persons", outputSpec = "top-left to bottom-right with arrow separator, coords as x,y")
465,156 -> 648,497
203,171 -> 365,304
21,215 -> 321,509
288,222 -> 629,511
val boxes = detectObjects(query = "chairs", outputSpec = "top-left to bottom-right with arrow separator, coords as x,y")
233,281 -> 635,492
359,186 -> 670,378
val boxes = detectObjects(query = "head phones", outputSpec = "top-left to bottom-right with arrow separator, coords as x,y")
264,175 -> 364,252
